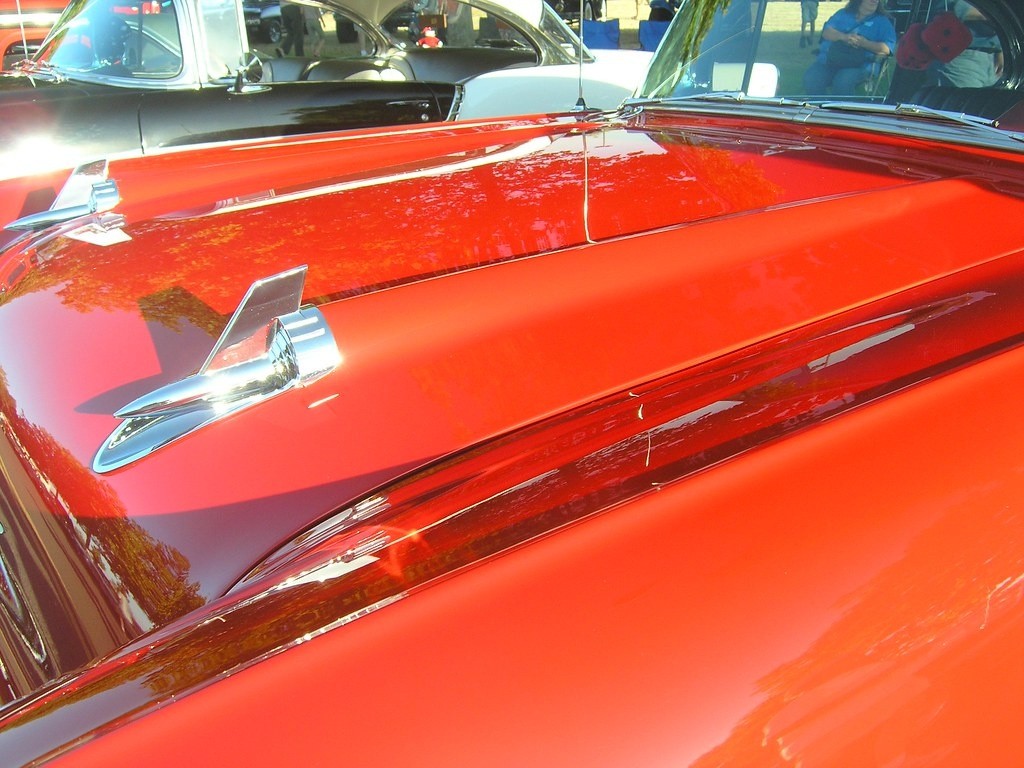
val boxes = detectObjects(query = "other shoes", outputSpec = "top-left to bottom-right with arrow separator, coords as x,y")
808,36 -> 813,45
800,35 -> 806,47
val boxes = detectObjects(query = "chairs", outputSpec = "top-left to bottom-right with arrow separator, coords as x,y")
580,17 -> 620,50
299,60 -> 387,81
257,59 -> 312,82
811,15 -> 898,103
637,20 -> 673,53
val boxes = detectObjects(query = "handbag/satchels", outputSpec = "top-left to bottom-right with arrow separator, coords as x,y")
827,41 -> 863,68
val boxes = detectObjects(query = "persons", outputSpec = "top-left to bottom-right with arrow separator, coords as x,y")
275,0 -> 326,58
801,0 -> 819,47
649,0 -> 682,22
802,0 -> 898,100
925,0 -> 1003,88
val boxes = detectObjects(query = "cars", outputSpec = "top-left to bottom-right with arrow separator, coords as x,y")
0,0 -> 1024,768
0,0 -> 781,172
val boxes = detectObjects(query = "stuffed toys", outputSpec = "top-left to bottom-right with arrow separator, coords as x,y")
415,27 -> 443,49
